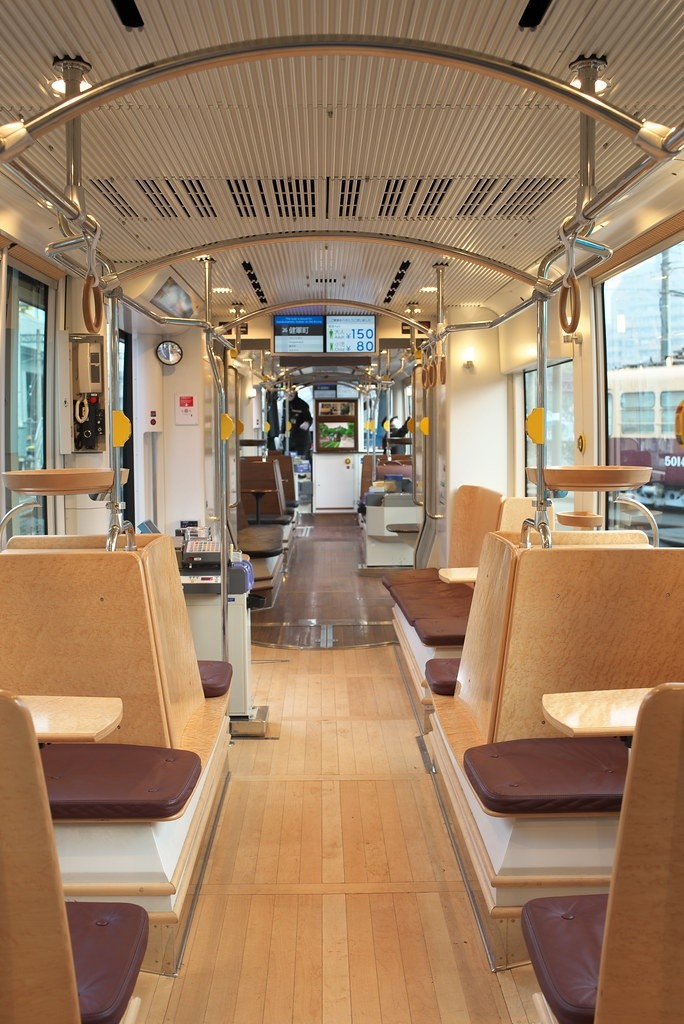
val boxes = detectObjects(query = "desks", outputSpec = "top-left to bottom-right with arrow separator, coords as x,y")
438,567 -> 477,584
542,687 -> 654,738
241,488 -> 277,524
17,695 -> 123,748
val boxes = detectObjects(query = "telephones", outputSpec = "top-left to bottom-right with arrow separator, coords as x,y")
78,343 -> 102,393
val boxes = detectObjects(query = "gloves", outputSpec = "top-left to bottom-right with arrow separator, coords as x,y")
279,434 -> 284,441
405,417 -> 411,427
299,422 -> 309,430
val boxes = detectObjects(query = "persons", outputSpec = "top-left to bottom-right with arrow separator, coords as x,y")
381,414 -> 412,458
279,387 -> 313,461
548,405 -> 574,434
329,403 -> 349,415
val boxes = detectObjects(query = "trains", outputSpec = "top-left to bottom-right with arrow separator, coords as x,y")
608,348 -> 684,506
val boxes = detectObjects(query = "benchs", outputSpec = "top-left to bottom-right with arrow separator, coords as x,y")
0,535 -> 231,1024
226,451 -> 300,591
375,483 -> 684,1024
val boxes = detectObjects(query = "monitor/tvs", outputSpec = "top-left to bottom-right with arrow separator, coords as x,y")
270,310 -> 378,357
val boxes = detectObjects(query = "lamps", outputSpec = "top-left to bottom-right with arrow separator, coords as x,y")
156,341 -> 183,365
